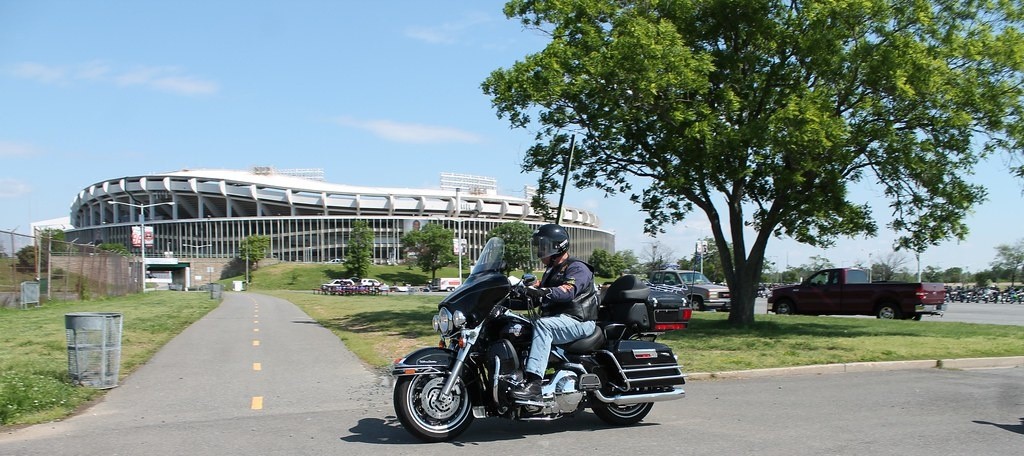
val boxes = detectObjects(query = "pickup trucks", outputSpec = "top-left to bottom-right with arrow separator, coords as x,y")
766,266 -> 948,321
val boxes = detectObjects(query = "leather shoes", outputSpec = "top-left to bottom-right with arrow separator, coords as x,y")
512,383 -> 543,400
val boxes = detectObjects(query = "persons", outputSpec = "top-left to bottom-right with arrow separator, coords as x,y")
357,276 -> 362,283
768,284 -> 772,291
503,224 -> 598,401
413,220 -> 420,231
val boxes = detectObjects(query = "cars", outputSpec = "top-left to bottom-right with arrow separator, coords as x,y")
329,259 -> 347,264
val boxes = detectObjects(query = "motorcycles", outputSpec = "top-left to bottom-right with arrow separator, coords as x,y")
393,236 -> 699,443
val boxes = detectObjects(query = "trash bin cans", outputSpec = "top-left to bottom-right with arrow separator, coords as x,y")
209,283 -> 221,299
63,311 -> 125,390
409,287 -> 415,295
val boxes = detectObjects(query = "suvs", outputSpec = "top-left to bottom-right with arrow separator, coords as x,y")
321,277 -> 358,292
650,270 -> 732,312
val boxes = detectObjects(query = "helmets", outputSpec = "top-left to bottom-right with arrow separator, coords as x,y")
531,224 -> 569,258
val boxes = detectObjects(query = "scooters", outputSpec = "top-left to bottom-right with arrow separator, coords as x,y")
944,284 -> 1024,304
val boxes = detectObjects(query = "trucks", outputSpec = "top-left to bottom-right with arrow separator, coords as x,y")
431,278 -> 460,292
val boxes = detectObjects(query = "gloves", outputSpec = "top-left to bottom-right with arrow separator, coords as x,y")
526,286 -> 552,306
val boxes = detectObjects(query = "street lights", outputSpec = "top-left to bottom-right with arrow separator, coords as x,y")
183,244 -> 213,291
107,201 -> 176,294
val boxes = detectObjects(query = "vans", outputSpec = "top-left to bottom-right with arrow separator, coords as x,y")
360,278 -> 381,286
